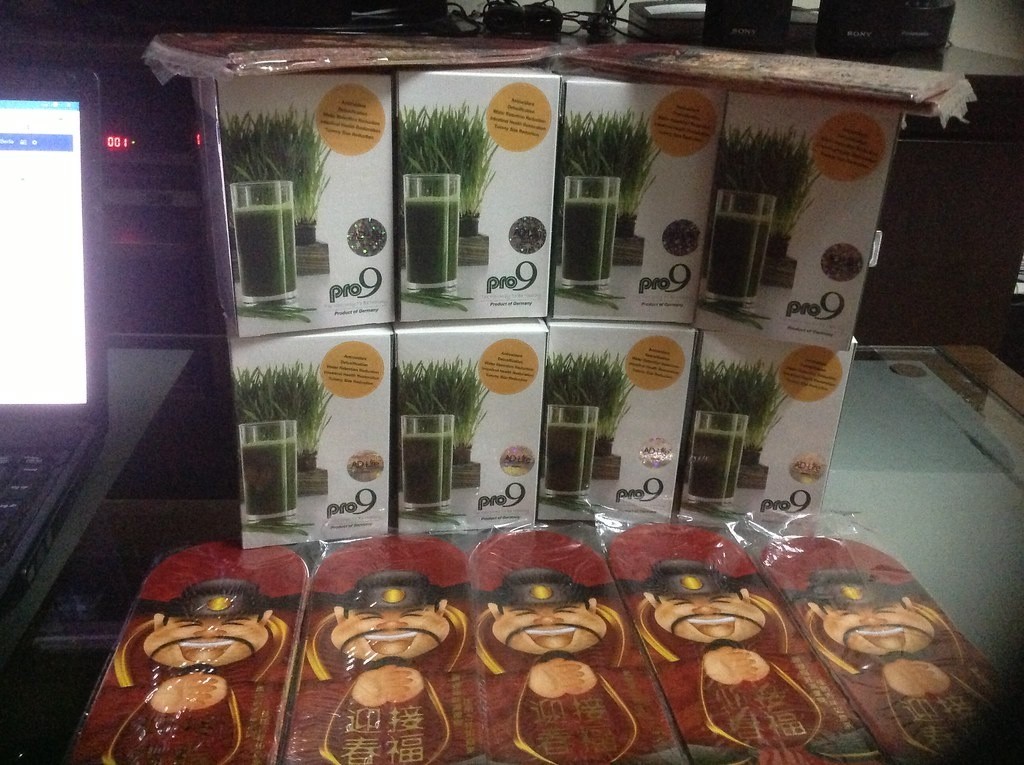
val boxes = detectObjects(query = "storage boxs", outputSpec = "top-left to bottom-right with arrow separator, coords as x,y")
681,335 -> 858,520
224,326 -> 392,551
696,93 -> 907,351
389,317 -> 547,536
553,70 -> 727,323
193,69 -> 397,336
537,324 -> 695,525
397,67 -> 562,326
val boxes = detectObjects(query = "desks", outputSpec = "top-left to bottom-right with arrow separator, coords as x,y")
0,344 -> 1024,765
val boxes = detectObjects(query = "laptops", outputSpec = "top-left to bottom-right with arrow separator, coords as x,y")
0,65 -> 108,621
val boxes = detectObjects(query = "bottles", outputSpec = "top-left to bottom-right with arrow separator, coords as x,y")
701,188 -> 777,309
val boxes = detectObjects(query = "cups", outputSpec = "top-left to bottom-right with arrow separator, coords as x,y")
403,173 -> 462,296
238,421 -> 297,525
563,176 -> 620,289
231,182 -> 298,307
545,404 -> 599,499
401,414 -> 456,515
686,410 -> 748,506
